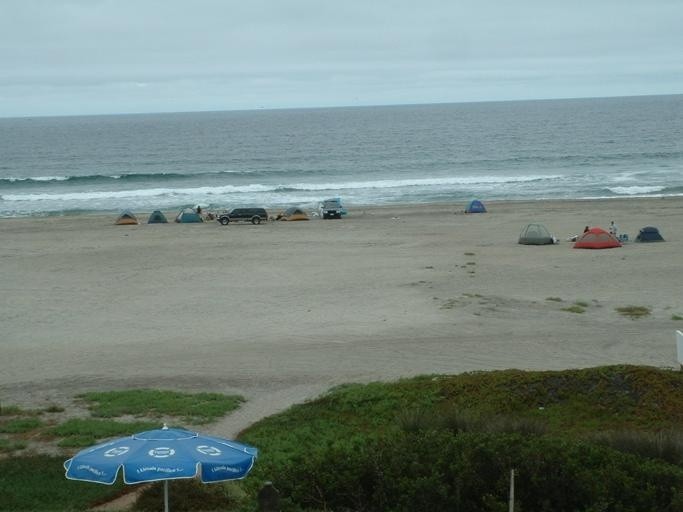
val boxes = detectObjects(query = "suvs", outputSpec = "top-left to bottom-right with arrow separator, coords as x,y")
217,208 -> 268,225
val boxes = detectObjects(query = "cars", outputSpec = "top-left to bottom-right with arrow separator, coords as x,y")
320,201 -> 342,219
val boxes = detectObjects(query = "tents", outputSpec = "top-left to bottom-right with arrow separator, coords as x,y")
146,211 -> 167,224
174,207 -> 203,224
462,199 -> 486,214
518,222 -> 559,246
570,228 -> 624,251
633,225 -> 664,242
112,209 -> 138,225
277,206 -> 311,221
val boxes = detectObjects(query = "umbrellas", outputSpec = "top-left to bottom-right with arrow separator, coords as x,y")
62,421 -> 260,511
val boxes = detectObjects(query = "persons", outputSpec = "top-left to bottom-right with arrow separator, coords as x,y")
196,205 -> 201,214
583,226 -> 589,233
607,221 -> 616,238
